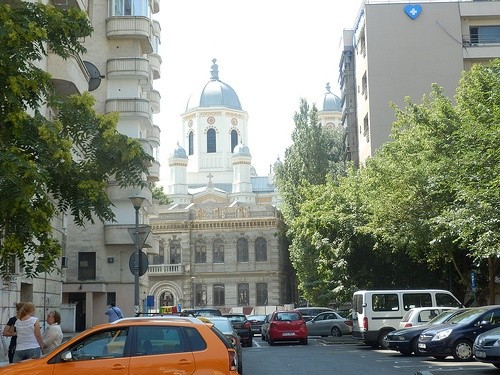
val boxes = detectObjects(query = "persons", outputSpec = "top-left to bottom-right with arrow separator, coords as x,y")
12,301 -> 44,363
2,302 -> 25,364
42,311 -> 64,355
104,302 -> 125,323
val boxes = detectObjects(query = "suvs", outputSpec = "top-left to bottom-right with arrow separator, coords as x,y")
222,313 -> 253,346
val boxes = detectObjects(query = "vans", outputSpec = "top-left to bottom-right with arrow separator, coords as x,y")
352,289 -> 466,351
291,307 -> 335,322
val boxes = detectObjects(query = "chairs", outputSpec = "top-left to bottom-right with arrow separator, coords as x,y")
143,339 -> 152,354
235,316 -> 241,320
385,304 -> 392,310
290,315 -> 297,320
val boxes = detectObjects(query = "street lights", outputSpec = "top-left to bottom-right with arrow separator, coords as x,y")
128,193 -> 146,317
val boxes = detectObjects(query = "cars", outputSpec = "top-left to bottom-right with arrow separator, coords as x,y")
261,310 -> 308,345
472,327 -> 500,370
11,310 -> 240,375
417,305 -> 500,362
399,307 -> 459,328
246,315 -> 269,337
386,308 -> 471,356
306,310 -> 353,338
103,308 -> 244,375
